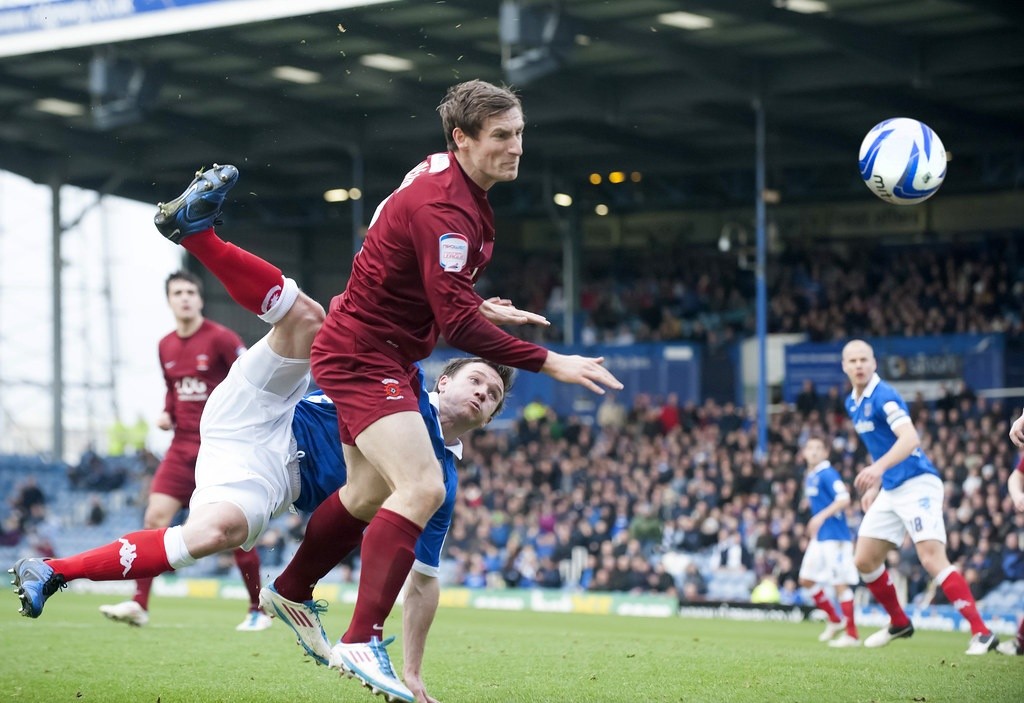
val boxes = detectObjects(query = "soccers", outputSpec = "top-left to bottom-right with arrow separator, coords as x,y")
858,117 -> 947,205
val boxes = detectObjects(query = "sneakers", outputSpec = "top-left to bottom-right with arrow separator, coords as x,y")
818,618 -> 848,641
995,639 -> 1020,654
98,600 -> 148,627
328,631 -> 414,703
153,163 -> 239,246
829,634 -> 860,647
7,557 -> 67,619
864,619 -> 914,648
257,576 -> 335,667
235,610 -> 273,631
964,630 -> 999,656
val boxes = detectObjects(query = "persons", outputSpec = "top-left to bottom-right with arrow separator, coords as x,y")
99,271 -> 273,631
798,435 -> 860,648
996,412 -> 1024,656
262,513 -> 354,583
0,479 -> 47,545
839,340 -> 999,655
68,446 -> 123,493
260,78 -> 624,703
7,165 -> 516,703
767,255 -> 1024,344
581,262 -> 756,357
908,381 -> 1024,604
441,377 -> 874,607
